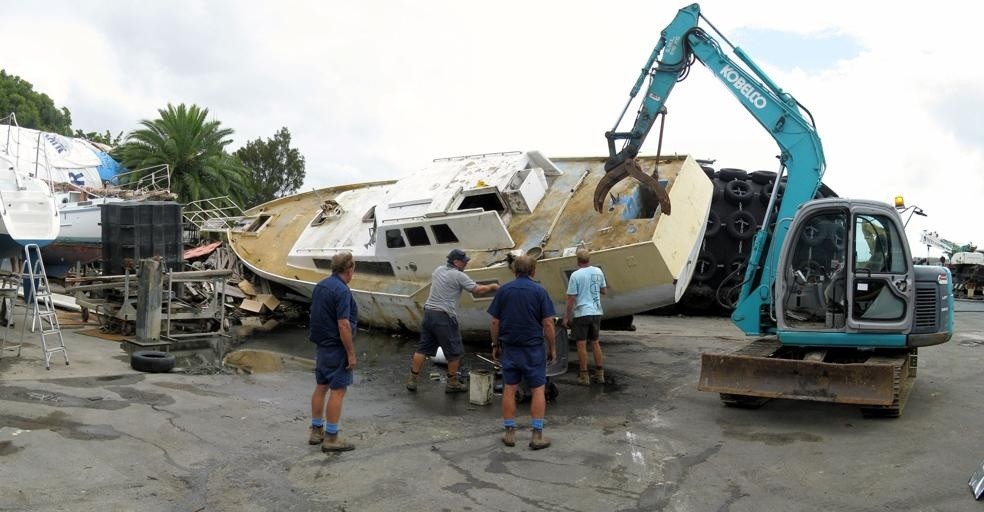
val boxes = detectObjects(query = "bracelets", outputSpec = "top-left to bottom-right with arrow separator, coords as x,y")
491,342 -> 500,349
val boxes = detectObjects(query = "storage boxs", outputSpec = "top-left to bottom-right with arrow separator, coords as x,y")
238,279 -> 281,313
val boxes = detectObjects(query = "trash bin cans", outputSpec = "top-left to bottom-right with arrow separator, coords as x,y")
0,288 -> 17,328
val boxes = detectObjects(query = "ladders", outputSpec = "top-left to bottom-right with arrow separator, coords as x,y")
0,244 -> 70,371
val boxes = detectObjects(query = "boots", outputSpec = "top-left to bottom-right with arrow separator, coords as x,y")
308,422 -> 324,444
527,429 -> 550,452
321,431 -> 354,452
446,375 -> 468,394
591,369 -> 606,385
500,427 -> 515,448
406,370 -> 419,392
575,371 -> 590,386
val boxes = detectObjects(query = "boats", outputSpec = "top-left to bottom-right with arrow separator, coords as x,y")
0,112 -> 171,267
181,150 -> 714,341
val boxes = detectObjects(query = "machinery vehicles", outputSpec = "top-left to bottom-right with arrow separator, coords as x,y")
594,3 -> 954,420
920,230 -> 984,302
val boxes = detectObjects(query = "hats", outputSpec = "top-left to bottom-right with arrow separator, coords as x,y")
447,249 -> 471,265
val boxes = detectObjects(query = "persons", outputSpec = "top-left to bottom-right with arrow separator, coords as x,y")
306,250 -> 358,450
560,247 -> 608,386
403,248 -> 502,395
485,256 -> 558,450
